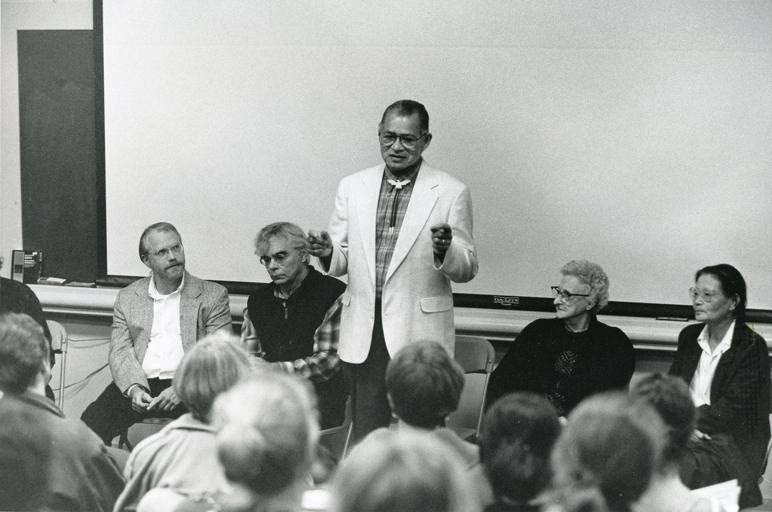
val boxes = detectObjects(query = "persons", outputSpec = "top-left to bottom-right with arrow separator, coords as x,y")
483,259 -> 637,421
78,221 -> 235,443
667,263 -> 771,504
0,311 -> 764,512
0,254 -> 56,407
304,99 -> 479,435
240,221 -> 349,428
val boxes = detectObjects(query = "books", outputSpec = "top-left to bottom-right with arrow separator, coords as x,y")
11,249 -> 43,284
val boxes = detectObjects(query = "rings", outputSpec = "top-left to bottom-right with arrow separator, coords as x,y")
442,239 -> 446,245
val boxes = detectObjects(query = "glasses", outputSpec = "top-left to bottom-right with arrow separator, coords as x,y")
688,287 -> 720,303
148,244 -> 182,257
551,286 -> 590,302
380,129 -> 427,149
260,251 -> 293,265
